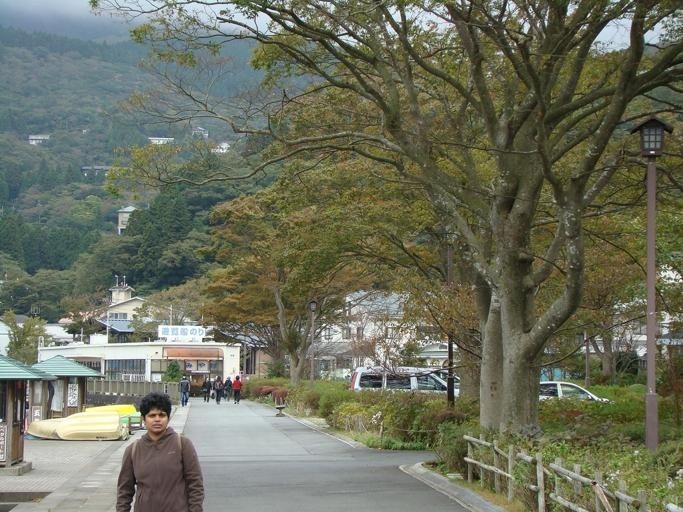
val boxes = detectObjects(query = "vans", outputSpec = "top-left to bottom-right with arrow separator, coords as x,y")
349,365 -> 460,397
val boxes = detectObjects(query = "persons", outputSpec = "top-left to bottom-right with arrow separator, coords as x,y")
230,375 -> 243,405
222,377 -> 232,402
213,376 -> 226,405
179,376 -> 192,408
200,377 -> 212,402
115,390 -> 206,511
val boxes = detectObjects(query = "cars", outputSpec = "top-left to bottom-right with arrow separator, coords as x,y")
538,380 -> 610,403
